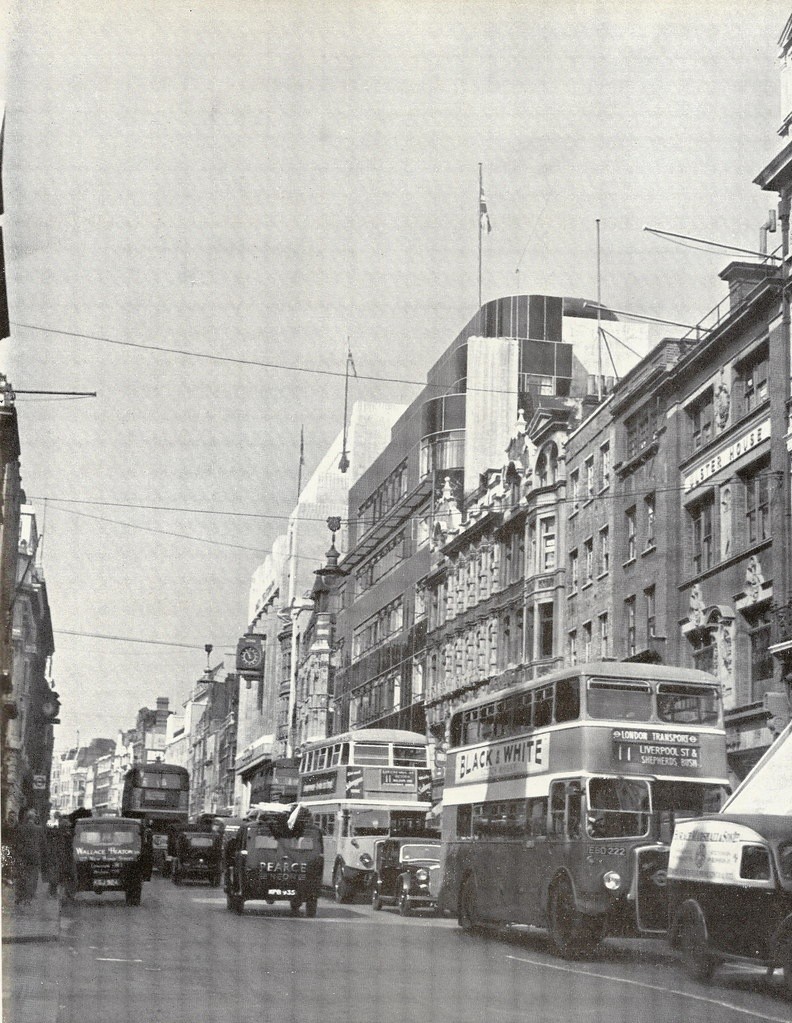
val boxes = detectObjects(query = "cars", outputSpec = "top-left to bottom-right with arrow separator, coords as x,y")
63,818 -> 146,909
367,837 -> 444,917
202,816 -> 250,863
163,823 -> 221,890
661,814 -> 791,1001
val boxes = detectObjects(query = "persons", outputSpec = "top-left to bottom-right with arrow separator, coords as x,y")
1,808 -> 73,905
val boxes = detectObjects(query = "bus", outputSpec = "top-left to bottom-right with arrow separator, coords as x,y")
298,728 -> 438,905
430,662 -> 729,960
98,808 -> 120,819
120,764 -> 191,868
248,757 -> 301,808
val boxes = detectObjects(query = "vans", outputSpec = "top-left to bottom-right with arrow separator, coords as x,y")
224,814 -> 322,917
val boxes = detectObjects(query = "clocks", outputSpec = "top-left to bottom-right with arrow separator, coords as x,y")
239,644 -> 261,668
